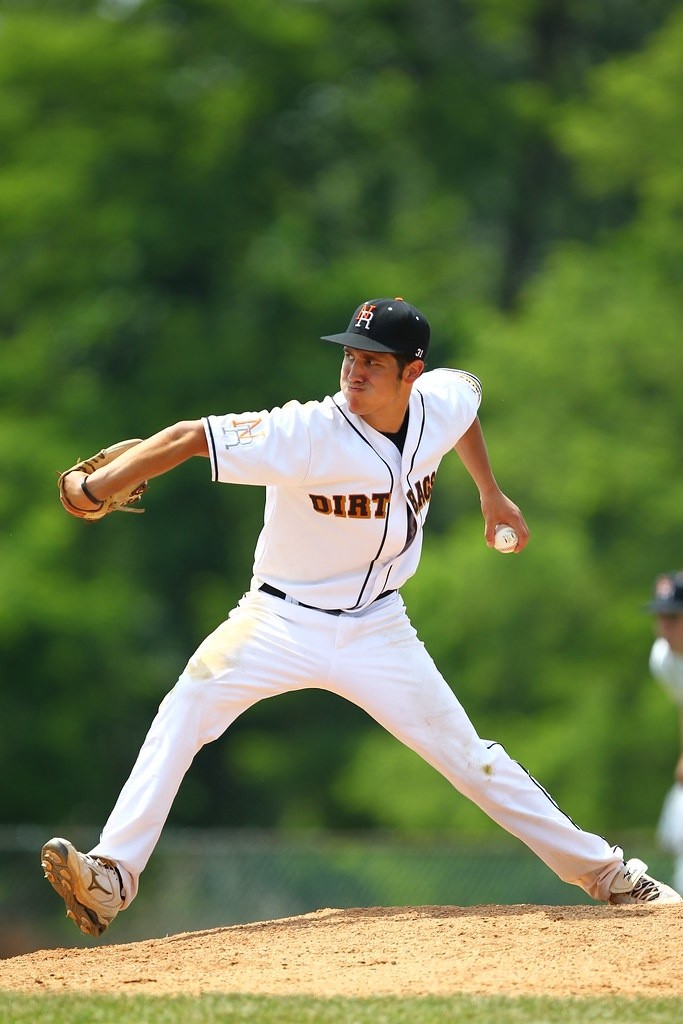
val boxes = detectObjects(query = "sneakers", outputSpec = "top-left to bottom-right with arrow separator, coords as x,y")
41,837 -> 127,938
608,858 -> 683,904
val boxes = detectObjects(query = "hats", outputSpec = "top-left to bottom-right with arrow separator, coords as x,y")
641,570 -> 683,614
319,299 -> 431,363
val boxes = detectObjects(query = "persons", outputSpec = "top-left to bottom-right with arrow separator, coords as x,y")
41,298 -> 683,936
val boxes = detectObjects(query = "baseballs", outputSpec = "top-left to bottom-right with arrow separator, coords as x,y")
491,524 -> 519,554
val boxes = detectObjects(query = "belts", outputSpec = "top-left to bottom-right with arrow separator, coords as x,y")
259,583 -> 396,616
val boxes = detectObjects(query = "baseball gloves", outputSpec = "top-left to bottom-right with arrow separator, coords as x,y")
54,438 -> 152,522
